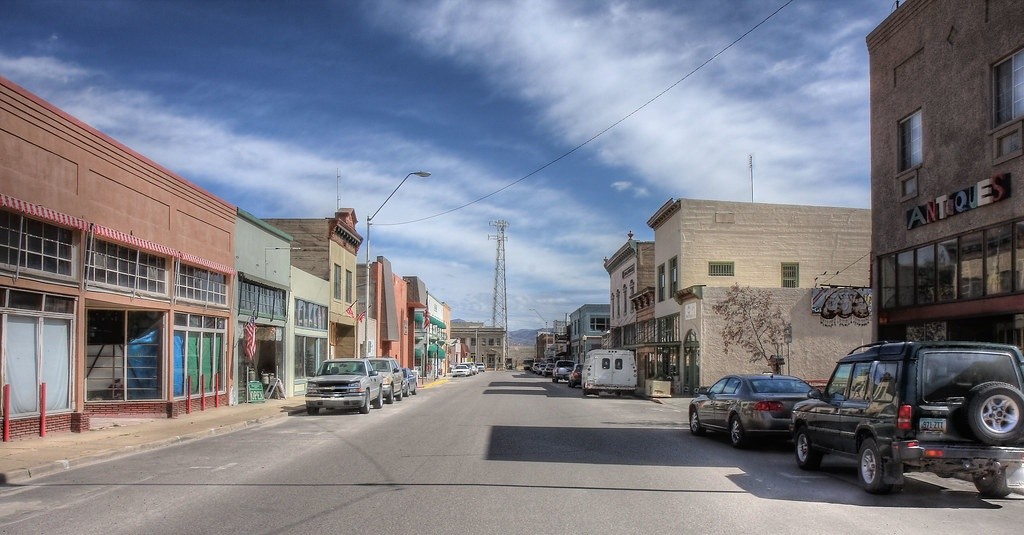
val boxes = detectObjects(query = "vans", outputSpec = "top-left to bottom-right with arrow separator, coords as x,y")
580,349 -> 639,397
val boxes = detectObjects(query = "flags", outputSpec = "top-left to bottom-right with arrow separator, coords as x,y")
346,307 -> 355,319
358,311 -> 365,323
422,292 -> 430,329
243,310 -> 256,361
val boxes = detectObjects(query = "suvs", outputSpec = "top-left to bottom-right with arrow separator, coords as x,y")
789,340 -> 1024,496
364,355 -> 405,404
304,357 -> 384,414
552,360 -> 577,383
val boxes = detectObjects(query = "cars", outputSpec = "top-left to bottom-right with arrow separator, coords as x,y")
400,367 -> 418,397
567,364 -> 584,388
475,362 -> 486,373
451,364 -> 471,377
462,362 -> 479,376
688,373 -> 816,449
543,362 -> 556,377
523,359 -> 546,375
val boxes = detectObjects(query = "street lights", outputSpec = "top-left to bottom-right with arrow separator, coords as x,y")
530,306 -> 548,357
363,172 -> 435,362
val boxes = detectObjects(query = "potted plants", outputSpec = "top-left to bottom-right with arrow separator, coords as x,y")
643,372 -> 672,398
